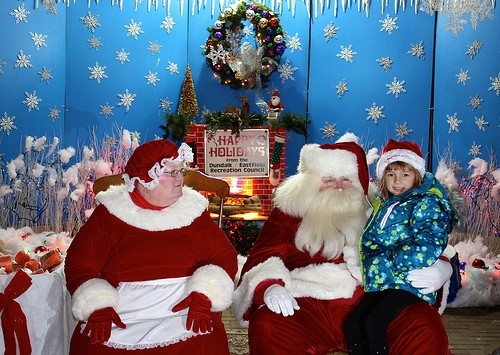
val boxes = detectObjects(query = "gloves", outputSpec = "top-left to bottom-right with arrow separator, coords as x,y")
409,259 -> 452,294
265,284 -> 300,317
84,306 -> 126,346
171,291 -> 215,334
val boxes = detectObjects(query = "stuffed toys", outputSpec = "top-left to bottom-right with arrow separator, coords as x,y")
267,89 -> 284,111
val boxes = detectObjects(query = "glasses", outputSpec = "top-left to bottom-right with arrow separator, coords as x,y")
162,168 -> 187,178
323,178 -> 352,187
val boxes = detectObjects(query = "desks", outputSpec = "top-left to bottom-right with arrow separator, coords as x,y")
0,258 -> 76,355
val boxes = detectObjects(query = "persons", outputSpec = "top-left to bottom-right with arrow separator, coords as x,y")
65,139 -> 239,355
344,138 -> 455,355
231,132 -> 453,355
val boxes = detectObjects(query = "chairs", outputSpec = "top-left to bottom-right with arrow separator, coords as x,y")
94,170 -> 230,227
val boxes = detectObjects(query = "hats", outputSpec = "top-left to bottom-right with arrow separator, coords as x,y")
297,132 -> 370,198
373,138 -> 426,178
121,139 -> 194,192
